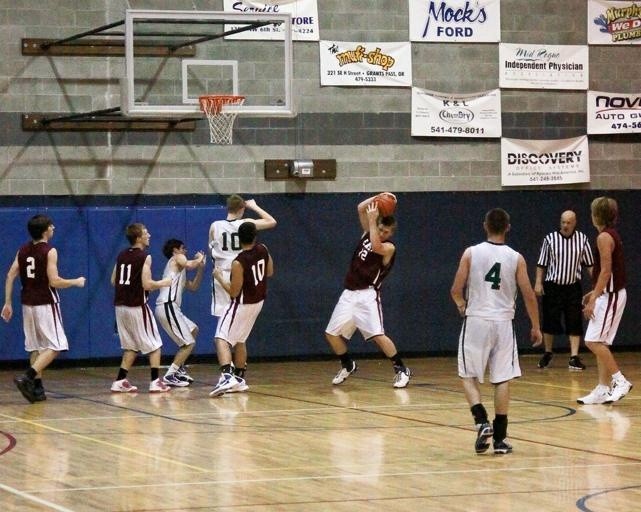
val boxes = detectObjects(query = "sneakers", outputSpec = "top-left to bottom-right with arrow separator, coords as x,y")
568,357 -> 586,369
494,438 -> 513,453
393,367 -> 413,388
14,365 -> 249,404
606,374 -> 634,402
332,360 -> 358,384
536,354 -> 553,368
578,385 -> 612,405
476,420 -> 495,453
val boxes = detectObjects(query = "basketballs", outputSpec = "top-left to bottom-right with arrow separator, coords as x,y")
372,196 -> 394,217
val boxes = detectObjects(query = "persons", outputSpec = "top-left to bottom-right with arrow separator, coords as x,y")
577,197 -> 632,404
111,222 -> 172,392
155,239 -> 206,386
0,215 -> 87,404
325,192 -> 411,388
534,210 -> 594,370
208,194 -> 278,374
208,222 -> 274,398
451,209 -> 543,454
